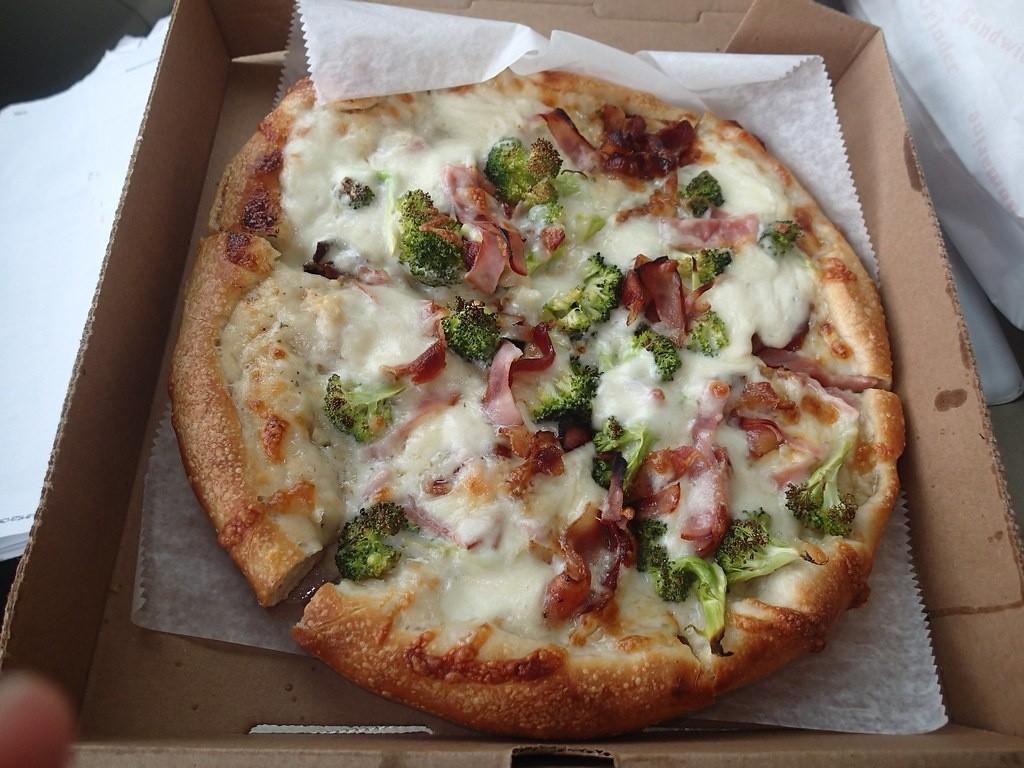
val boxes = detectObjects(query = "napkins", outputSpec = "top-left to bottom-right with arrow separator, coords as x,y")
0,12 -> 171,561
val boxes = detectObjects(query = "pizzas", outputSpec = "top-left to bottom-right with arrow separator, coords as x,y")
169,65 -> 907,742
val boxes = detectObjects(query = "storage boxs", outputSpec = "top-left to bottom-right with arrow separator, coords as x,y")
0,0 -> 1024,768
